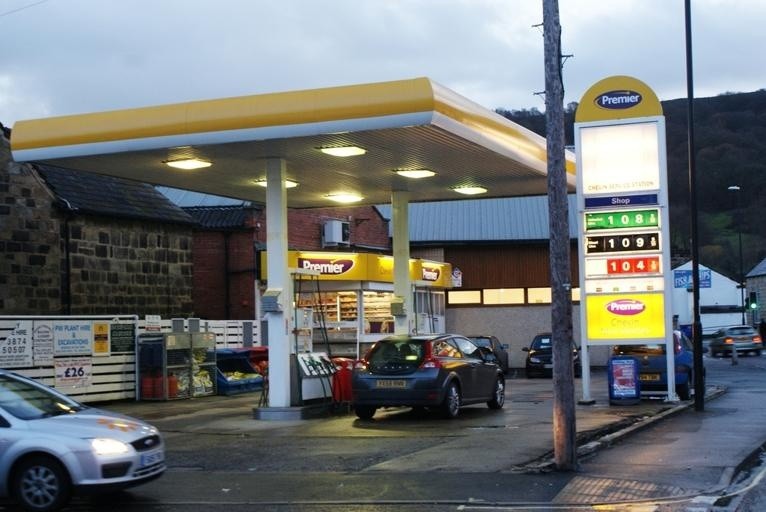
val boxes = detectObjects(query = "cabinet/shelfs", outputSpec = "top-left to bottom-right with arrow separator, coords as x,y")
136,333 -> 216,401
296,292 -> 358,322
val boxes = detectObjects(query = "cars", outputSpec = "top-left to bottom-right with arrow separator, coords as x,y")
710,325 -> 765,357
0,370 -> 167,508
522,333 -> 581,378
351,333 -> 508,418
607,330 -> 695,400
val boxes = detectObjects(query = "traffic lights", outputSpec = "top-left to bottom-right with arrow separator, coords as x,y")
749,292 -> 758,310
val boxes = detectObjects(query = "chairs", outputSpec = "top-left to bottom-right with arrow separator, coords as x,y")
399,344 -> 411,355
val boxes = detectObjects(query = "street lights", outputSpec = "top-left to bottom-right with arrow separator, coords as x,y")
728,186 -> 745,325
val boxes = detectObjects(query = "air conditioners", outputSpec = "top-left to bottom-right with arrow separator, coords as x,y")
324,221 -> 350,244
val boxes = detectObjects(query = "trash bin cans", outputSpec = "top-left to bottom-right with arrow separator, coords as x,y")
330,357 -> 356,401
